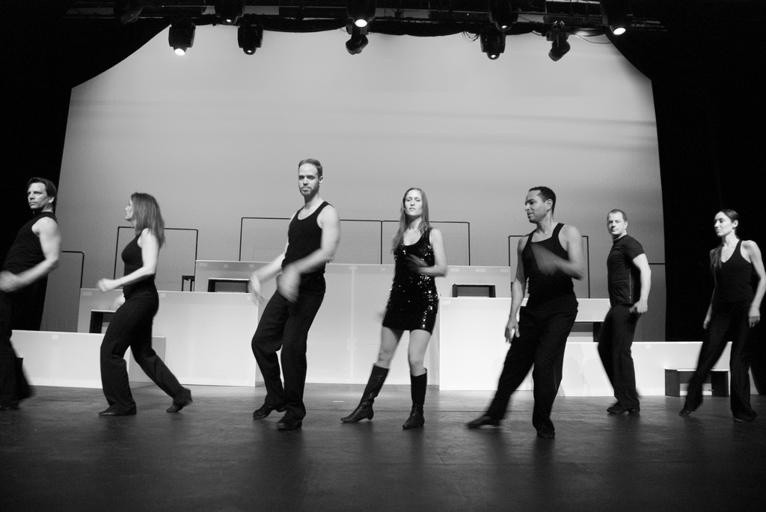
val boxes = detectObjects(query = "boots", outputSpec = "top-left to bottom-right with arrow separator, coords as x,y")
402,367 -> 427,429
341,364 -> 389,423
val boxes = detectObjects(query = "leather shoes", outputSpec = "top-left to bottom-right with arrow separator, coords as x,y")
679,409 -> 692,416
468,415 -> 501,429
167,405 -> 182,413
98,408 -> 117,416
537,432 -> 555,439
278,412 -> 306,423
253,403 -> 287,421
733,409 -> 756,421
606,402 -> 639,415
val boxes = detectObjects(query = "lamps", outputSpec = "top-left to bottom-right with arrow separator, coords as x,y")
479,24 -> 506,60
168,21 -> 196,56
598,1 -> 629,36
531,17 -> 570,61
238,14 -> 263,54
346,16 -> 369,55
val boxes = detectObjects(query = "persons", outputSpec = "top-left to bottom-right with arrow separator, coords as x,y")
248,158 -> 342,431
0,176 -> 62,410
96,192 -> 193,417
597,209 -> 652,416
465,186 -> 587,439
678,206 -> 765,424
341,187 -> 448,428
